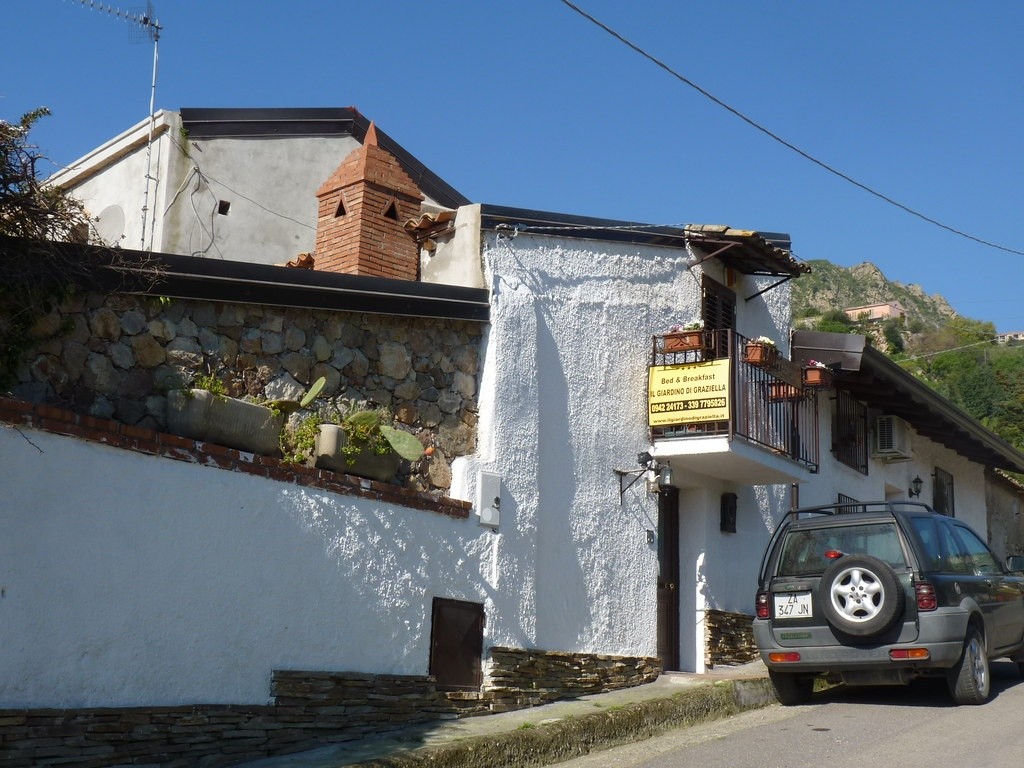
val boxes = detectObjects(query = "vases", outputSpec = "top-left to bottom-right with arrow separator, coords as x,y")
770,382 -> 805,400
663,332 -> 702,348
804,368 -> 834,390
744,343 -> 773,367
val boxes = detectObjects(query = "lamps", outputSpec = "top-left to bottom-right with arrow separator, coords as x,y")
612,462 -> 677,503
908,474 -> 923,499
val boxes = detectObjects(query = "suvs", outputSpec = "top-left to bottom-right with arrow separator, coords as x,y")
752,499 -> 1024,706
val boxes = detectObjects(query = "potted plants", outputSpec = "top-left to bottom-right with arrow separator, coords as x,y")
168,368 -> 327,453
286,406 -> 425,484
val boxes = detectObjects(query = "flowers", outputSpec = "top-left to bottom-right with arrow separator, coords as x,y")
669,319 -> 706,332
751,336 -> 776,345
808,359 -> 832,370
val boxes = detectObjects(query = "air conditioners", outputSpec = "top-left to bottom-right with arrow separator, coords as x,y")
876,416 -> 913,458
